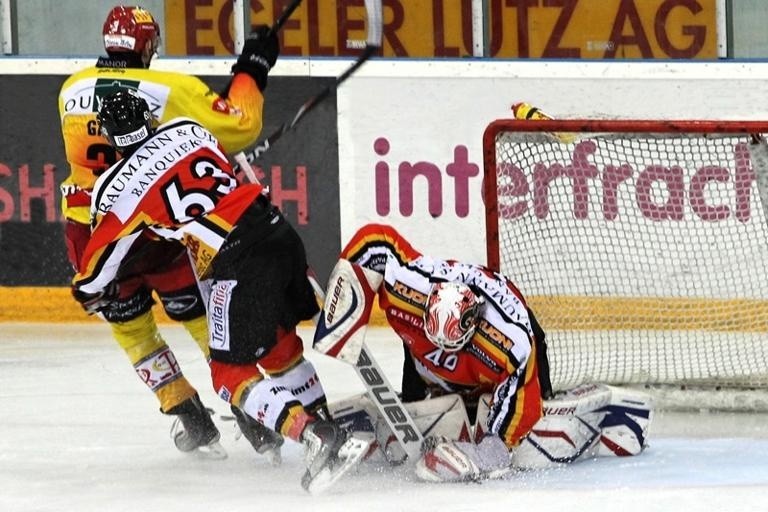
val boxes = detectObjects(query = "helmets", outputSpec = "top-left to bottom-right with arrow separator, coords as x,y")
103,5 -> 161,67
421,279 -> 487,355
95,91 -> 150,152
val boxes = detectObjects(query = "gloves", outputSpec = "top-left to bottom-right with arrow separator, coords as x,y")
412,434 -> 513,486
230,22 -> 280,90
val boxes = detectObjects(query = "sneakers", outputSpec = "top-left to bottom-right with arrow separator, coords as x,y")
230,404 -> 284,454
300,416 -> 348,492
173,392 -> 221,453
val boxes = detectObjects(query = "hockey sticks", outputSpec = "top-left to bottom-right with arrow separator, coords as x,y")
231,151 -> 424,464
233,0 -> 382,173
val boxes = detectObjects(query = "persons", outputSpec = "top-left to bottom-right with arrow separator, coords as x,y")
311,222 -> 651,483
55,5 -> 284,453
71,86 -> 348,489
